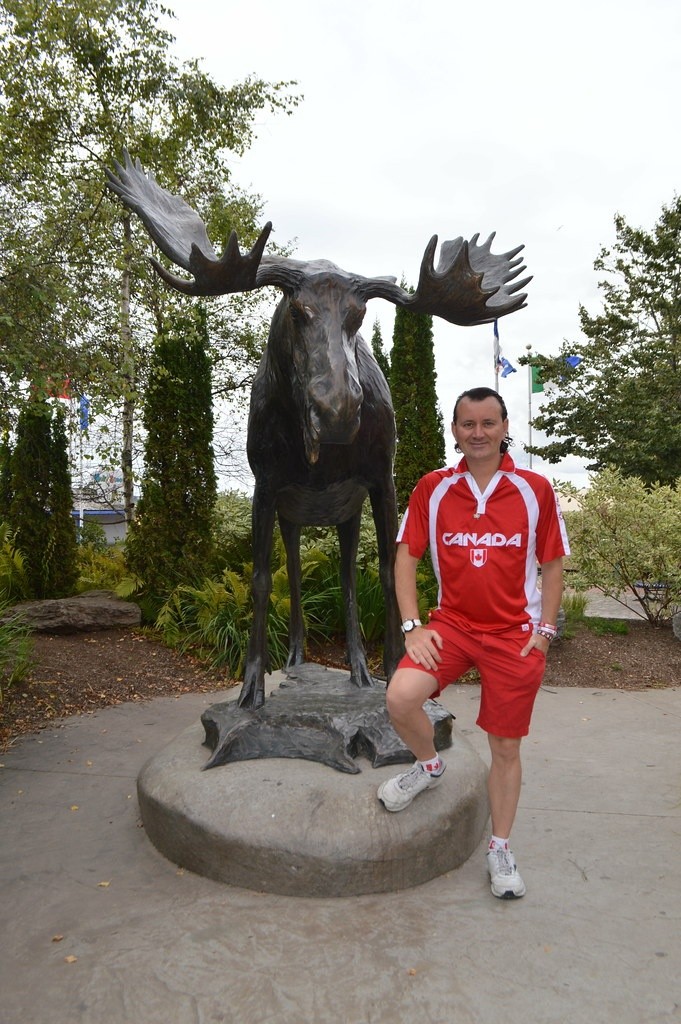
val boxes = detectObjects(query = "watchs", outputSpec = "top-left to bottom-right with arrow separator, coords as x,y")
400,619 -> 422,634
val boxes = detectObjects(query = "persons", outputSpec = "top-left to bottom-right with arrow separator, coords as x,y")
376,387 -> 571,899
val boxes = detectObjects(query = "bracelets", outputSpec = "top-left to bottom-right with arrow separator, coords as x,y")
537,622 -> 557,641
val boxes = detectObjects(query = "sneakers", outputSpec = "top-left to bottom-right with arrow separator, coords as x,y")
377,760 -> 448,813
485,840 -> 526,899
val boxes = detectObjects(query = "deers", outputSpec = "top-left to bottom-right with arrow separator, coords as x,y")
84,145 -> 535,710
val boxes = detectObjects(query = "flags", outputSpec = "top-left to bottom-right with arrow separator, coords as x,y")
26,351 -> 72,401
558,354 -> 582,383
78,394 -> 89,428
530,356 -> 544,393
494,319 -> 517,378
